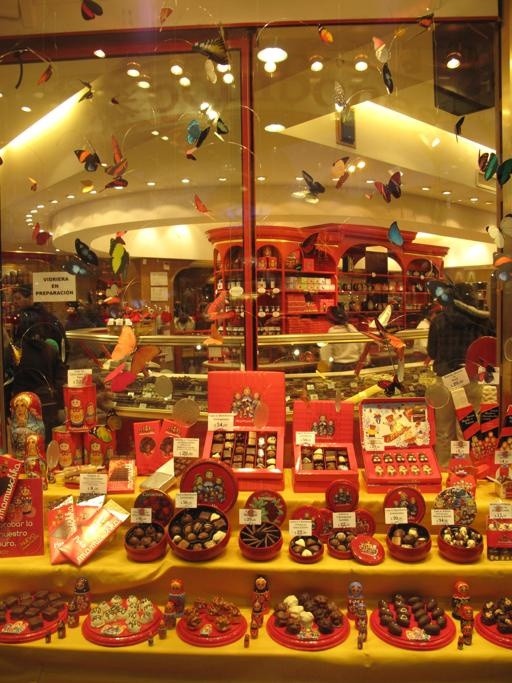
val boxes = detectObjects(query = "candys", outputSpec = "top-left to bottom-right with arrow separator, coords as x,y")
1,430 -> 512,643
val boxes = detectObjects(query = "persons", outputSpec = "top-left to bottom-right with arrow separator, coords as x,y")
192,302 -> 212,373
189,468 -> 227,503
175,306 -> 196,373
301,486 -> 418,534
313,303 -> 372,375
411,301 -> 445,362
1,283 -> 113,453
424,282 -> 496,470
229,385 -> 336,436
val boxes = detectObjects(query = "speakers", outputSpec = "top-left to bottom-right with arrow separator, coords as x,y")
339,108 -> 356,144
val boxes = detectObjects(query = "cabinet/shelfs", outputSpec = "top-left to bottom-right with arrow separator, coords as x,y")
206,219 -> 451,339
4,476 -> 512,679
